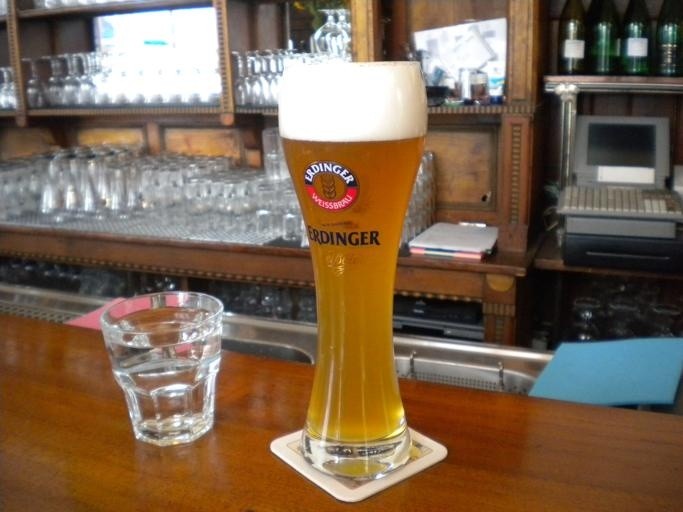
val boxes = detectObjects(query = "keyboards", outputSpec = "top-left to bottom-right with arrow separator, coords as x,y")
556,186 -> 683,222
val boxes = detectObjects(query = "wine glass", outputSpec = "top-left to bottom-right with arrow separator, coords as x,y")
3,134 -> 309,255
563,278 -> 682,338
0,2 -> 352,106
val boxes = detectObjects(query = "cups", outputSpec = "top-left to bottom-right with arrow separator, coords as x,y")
97,288 -> 229,451
275,57 -> 435,479
441,65 -> 467,106
469,72 -> 492,106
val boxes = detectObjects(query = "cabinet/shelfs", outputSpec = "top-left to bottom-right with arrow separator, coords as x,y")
534,75 -> 683,281
1,0 -> 552,346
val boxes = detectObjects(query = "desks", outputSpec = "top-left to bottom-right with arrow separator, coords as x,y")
1,316 -> 683,512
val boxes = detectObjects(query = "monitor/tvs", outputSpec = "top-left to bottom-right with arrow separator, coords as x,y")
572,114 -> 672,190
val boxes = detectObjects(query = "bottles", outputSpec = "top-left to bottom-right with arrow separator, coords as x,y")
621,0 -> 655,75
654,0 -> 683,75
585,0 -> 621,73
556,1 -> 586,74
